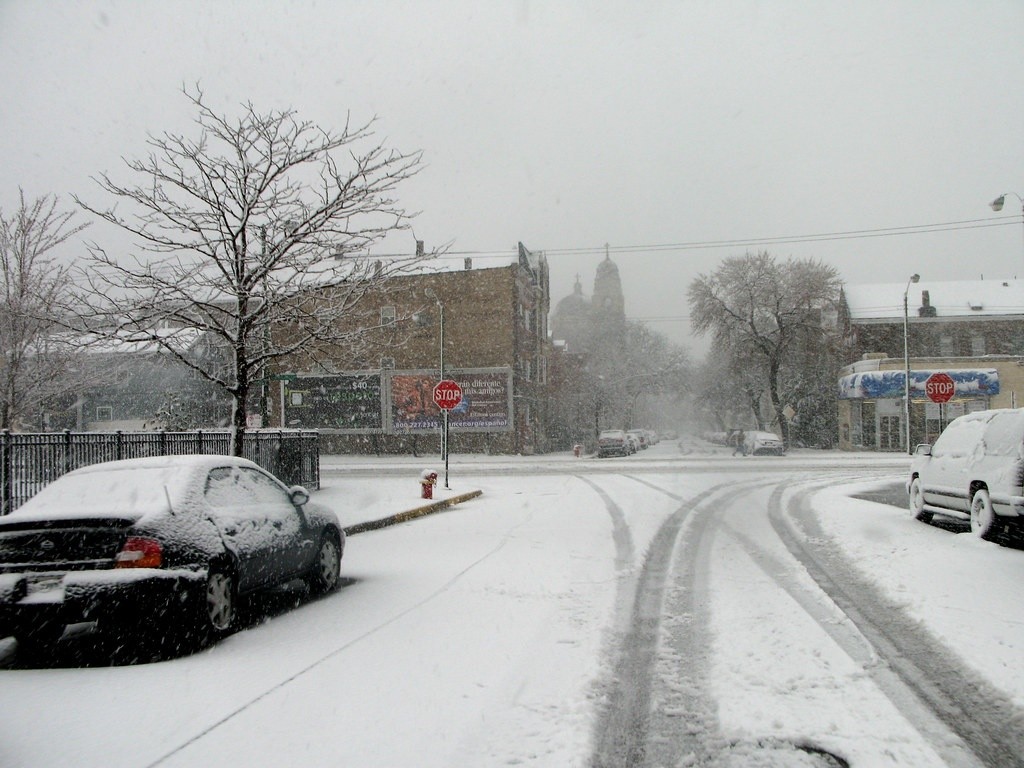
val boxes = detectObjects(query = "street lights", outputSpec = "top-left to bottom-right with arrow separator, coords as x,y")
423,288 -> 448,460
989,191 -> 1024,213
902,273 -> 920,459
259,223 -> 275,427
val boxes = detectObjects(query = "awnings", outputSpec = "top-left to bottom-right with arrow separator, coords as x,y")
838,368 -> 999,399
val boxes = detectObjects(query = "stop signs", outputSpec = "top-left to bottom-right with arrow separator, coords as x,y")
433,380 -> 467,414
925,372 -> 956,406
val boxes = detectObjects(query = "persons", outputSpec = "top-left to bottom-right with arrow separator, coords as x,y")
732,429 -> 746,456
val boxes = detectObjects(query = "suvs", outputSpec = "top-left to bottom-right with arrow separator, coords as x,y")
906,408 -> 1024,538
597,430 -> 631,458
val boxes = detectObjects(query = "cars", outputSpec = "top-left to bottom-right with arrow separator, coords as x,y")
705,429 -> 783,457
627,429 -> 660,448
1,454 -> 343,650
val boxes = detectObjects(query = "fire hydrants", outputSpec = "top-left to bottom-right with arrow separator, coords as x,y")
419,469 -> 440,500
